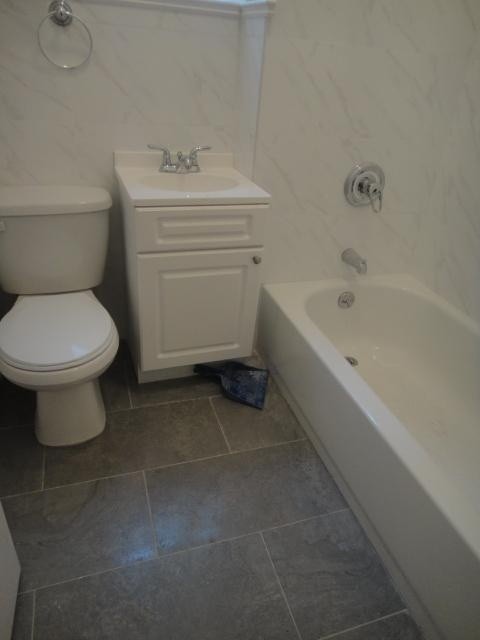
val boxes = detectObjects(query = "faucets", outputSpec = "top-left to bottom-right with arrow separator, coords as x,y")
177,144 -> 201,173
341,245 -> 370,275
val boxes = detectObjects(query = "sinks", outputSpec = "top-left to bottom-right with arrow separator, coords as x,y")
135,164 -> 251,200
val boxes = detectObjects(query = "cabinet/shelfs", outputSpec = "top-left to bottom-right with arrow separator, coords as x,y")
132,205 -> 269,372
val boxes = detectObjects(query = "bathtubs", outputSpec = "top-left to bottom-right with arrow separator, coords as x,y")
259,271 -> 480,638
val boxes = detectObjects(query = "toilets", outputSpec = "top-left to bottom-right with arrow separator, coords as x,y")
0,182 -> 119,448
